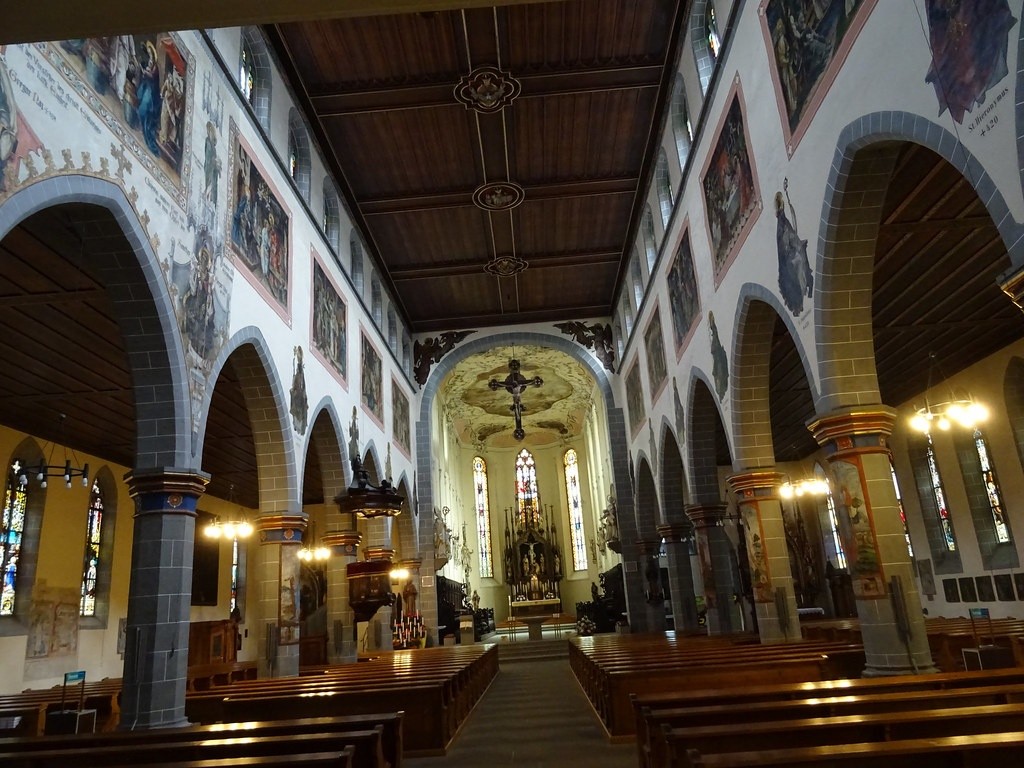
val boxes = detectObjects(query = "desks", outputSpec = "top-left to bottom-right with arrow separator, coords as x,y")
511,598 -> 560,641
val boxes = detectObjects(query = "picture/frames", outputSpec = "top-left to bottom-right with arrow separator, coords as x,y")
625,561 -> 637,572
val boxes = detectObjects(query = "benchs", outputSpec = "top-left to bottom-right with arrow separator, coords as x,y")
0,615 -> 1024,768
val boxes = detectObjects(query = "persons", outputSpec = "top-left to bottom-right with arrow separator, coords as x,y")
461,542 -> 474,568
402,578 -> 418,612
540,553 -> 545,573
590,539 -> 596,560
603,496 -> 618,541
471,590 -> 481,610
522,554 -> 529,576
554,555 -> 560,574
524,542 -> 539,558
590,581 -> 598,601
507,558 -> 512,579
434,517 -> 453,560
597,526 -> 606,552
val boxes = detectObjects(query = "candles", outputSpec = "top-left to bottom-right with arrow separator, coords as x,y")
417,609 -> 419,619
408,617 -> 409,625
400,634 -> 402,644
417,623 -> 419,632
401,610 -> 404,621
512,580 -> 553,596
421,617 -> 425,626
555,582 -> 557,591
414,618 -> 416,625
414,627 -> 415,638
399,629 -> 401,638
406,629 -> 408,639
395,625 -> 398,634
403,622 -> 405,633
410,622 -> 411,631
508,595 -> 511,605
408,632 -> 411,642
401,619 -> 403,626
420,627 -> 422,637
404,640 -> 407,647
556,586 -> 558,593
394,619 -> 396,627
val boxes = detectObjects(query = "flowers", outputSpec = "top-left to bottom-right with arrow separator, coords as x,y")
572,615 -> 596,634
546,592 -> 555,598
516,595 -> 525,601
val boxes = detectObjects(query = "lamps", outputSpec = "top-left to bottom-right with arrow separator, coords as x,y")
653,536 -> 667,559
201,482 -> 256,542
777,444 -> 830,505
19,402 -> 89,488
295,521 -> 333,564
905,352 -> 996,436
715,479 -> 743,527
681,529 -> 695,542
